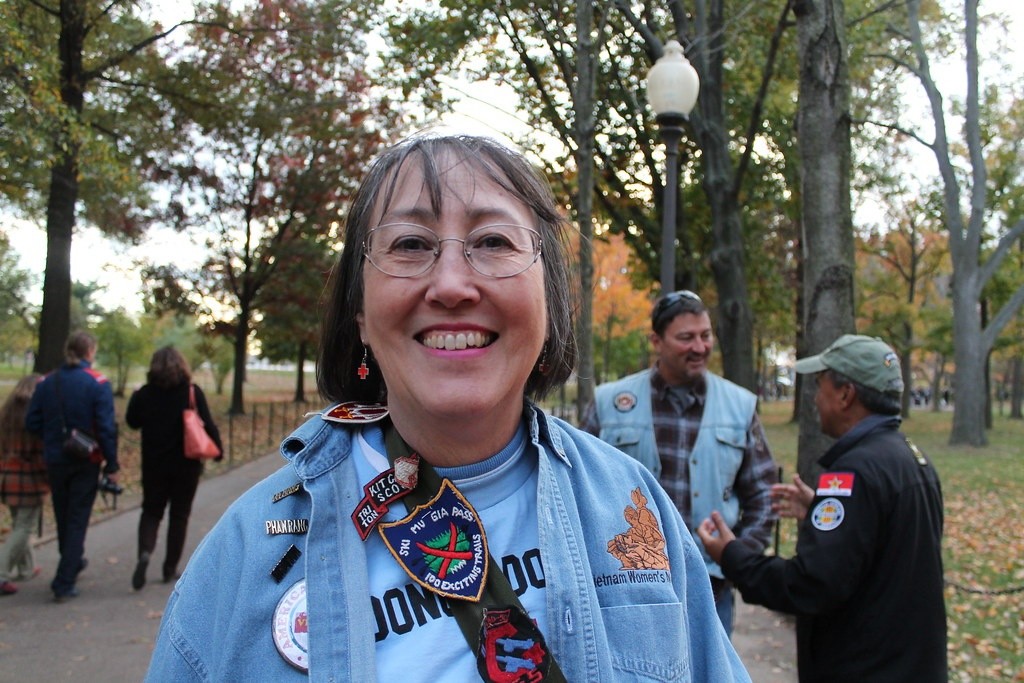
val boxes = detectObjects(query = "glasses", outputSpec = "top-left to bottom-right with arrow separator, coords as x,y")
357,224 -> 545,280
653,290 -> 702,320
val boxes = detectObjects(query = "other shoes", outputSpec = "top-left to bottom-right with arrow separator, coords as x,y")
164,562 -> 180,583
0,582 -> 17,594
133,553 -> 149,590
32,566 -> 39,577
56,588 -> 79,602
76,558 -> 88,572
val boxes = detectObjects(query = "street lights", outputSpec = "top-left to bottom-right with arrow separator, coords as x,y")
644,39 -> 699,293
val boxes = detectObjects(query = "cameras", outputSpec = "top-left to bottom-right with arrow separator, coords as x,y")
99,479 -> 127,497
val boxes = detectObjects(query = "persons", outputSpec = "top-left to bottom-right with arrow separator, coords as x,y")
915,388 -> 949,406
577,289 -> 780,641
125,347 -> 223,590
0,332 -> 119,599
145,135 -> 754,683
698,334 -> 948,683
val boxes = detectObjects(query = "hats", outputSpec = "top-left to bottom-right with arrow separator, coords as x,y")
793,333 -> 904,392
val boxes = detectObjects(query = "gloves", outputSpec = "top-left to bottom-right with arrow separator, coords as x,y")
213,446 -> 223,462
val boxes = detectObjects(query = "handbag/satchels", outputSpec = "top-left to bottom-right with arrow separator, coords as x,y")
185,383 -> 220,459
63,428 -> 104,466
